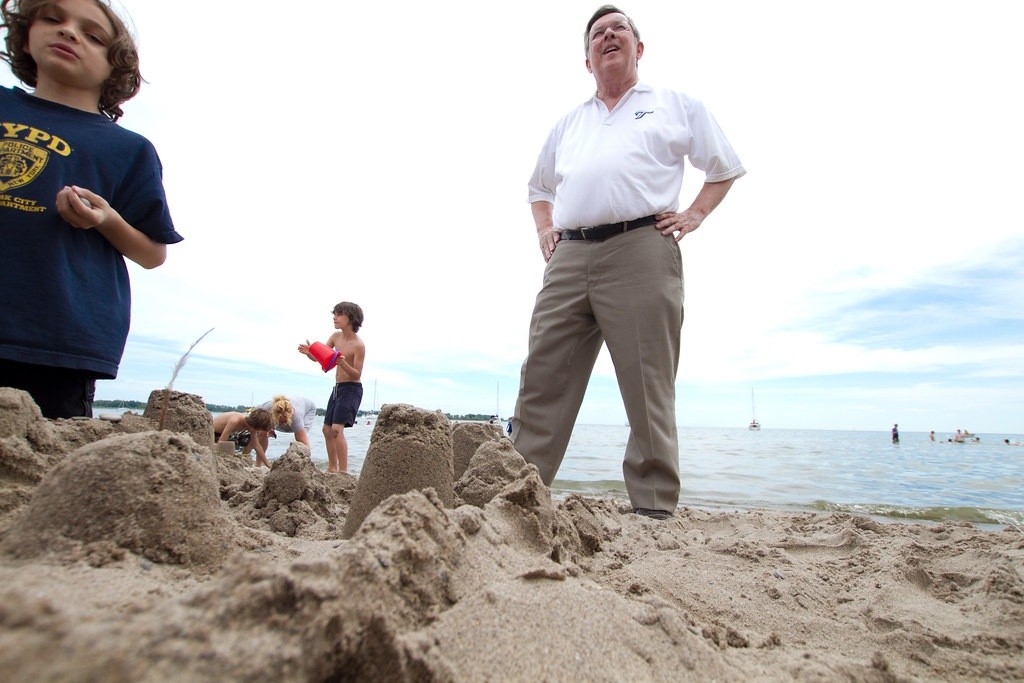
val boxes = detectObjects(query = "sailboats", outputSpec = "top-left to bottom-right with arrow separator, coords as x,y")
747,384 -> 760,431
366,377 -> 380,420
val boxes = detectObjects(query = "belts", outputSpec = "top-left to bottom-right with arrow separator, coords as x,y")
560,214 -> 660,240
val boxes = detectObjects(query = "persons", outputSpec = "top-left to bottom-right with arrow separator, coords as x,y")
0,0 -> 184,419
367,420 -> 371,425
299,302 -> 364,474
488,416 -> 514,437
213,394 -> 316,468
892,424 -> 900,443
1005,439 -> 1022,446
509,6 -> 746,521
929,429 -> 980,443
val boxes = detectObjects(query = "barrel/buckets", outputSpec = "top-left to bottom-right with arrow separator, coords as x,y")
309,341 -> 341,373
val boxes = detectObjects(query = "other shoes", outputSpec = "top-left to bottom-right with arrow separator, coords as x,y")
633,508 -> 671,520
